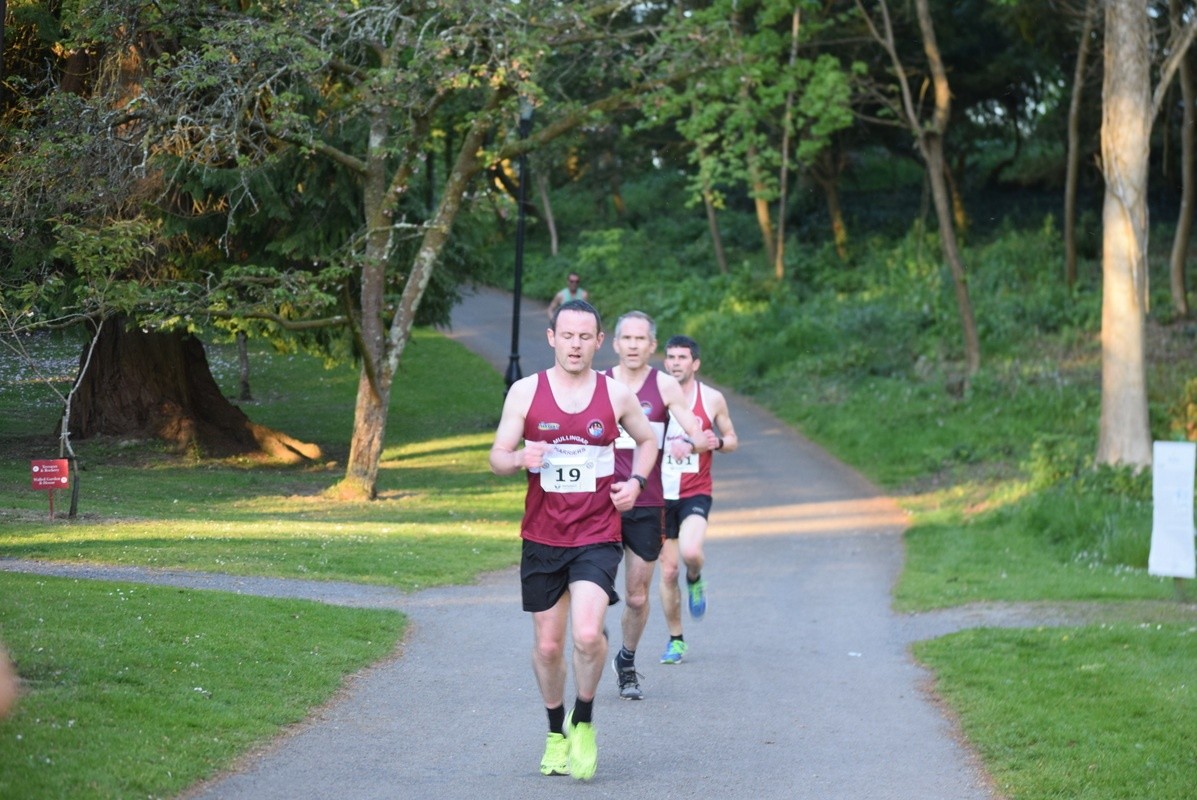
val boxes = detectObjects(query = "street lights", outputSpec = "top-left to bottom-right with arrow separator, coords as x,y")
505,95 -> 537,399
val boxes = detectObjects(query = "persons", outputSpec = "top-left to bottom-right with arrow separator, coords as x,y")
488,300 -> 739,783
548,273 -> 590,321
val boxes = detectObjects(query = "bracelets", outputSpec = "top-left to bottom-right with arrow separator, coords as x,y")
715,437 -> 724,451
683,438 -> 696,454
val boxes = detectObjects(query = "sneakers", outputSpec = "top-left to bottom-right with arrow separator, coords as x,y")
659,641 -> 684,663
688,581 -> 705,619
539,732 -> 571,775
612,651 -> 644,700
562,707 -> 598,781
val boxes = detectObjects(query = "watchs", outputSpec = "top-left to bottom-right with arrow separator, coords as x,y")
628,473 -> 647,488
